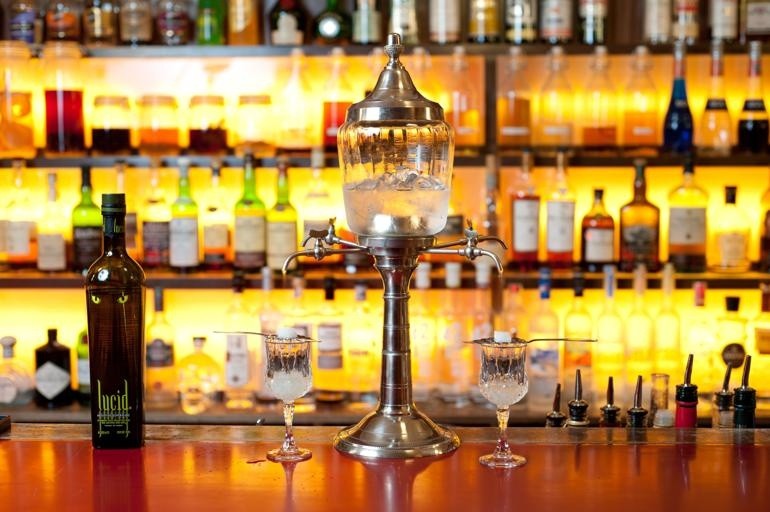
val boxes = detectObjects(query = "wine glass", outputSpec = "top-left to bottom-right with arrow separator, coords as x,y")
260,336 -> 313,463
476,337 -> 530,470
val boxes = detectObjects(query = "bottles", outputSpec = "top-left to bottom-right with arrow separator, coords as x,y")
1,154 -> 770,272
544,353 -> 760,426
13,0 -> 770,46
2,268 -> 770,414
0,40 -> 769,152
85,191 -> 145,450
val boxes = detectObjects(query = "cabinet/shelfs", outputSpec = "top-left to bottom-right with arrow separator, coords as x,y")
0,0 -> 767,426
